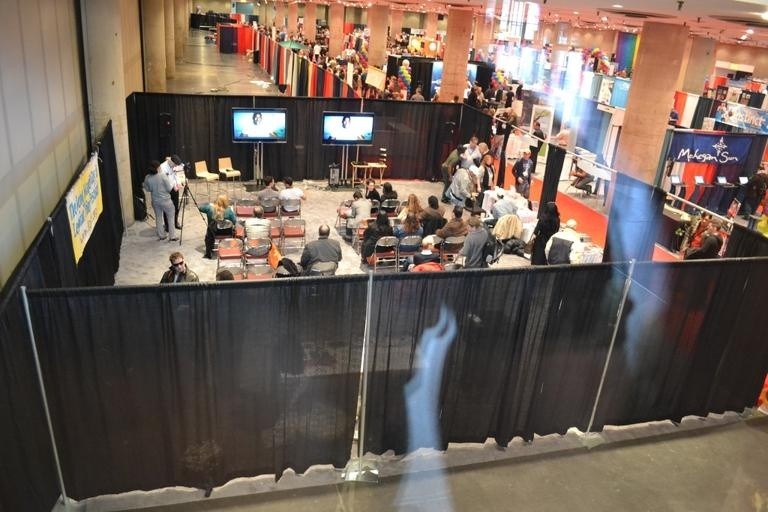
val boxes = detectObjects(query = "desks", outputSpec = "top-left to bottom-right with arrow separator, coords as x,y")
481,188 -> 538,249
350,159 -> 386,189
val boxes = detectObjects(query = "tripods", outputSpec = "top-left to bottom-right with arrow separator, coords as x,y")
176,179 -> 208,244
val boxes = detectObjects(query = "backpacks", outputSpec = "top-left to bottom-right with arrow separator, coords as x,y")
483,236 -> 504,264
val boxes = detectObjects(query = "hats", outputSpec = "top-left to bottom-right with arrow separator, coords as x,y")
523,149 -> 531,155
457,144 -> 467,151
171,154 -> 181,165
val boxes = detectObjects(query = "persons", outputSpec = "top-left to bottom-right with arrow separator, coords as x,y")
241,111 -> 276,136
530,200 -> 580,265
144,153 -> 341,285
343,179 -> 490,272
529,122 -> 547,172
330,115 -> 363,139
679,212 -> 723,260
611,54 -> 616,61
512,151 -> 534,198
450,70 -> 522,156
569,154 -> 610,195
442,137 -> 496,214
249,18 -> 439,103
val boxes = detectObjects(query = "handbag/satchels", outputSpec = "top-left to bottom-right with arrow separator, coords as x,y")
524,235 -> 534,254
518,178 -> 529,199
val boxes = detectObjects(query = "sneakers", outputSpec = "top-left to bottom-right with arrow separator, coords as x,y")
587,185 -> 597,196
202,253 -> 211,259
158,221 -> 182,241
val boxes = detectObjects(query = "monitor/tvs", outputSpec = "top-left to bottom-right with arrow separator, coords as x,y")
232,108 -> 288,144
322,111 -> 374,146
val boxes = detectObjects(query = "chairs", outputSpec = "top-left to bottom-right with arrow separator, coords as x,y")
193,160 -> 220,207
335,199 -> 506,268
309,261 -> 338,277
217,158 -> 243,202
214,197 -> 307,279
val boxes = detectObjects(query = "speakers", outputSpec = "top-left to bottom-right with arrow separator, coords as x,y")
442,121 -> 455,144
328,166 -> 340,188
159,114 -> 172,137
